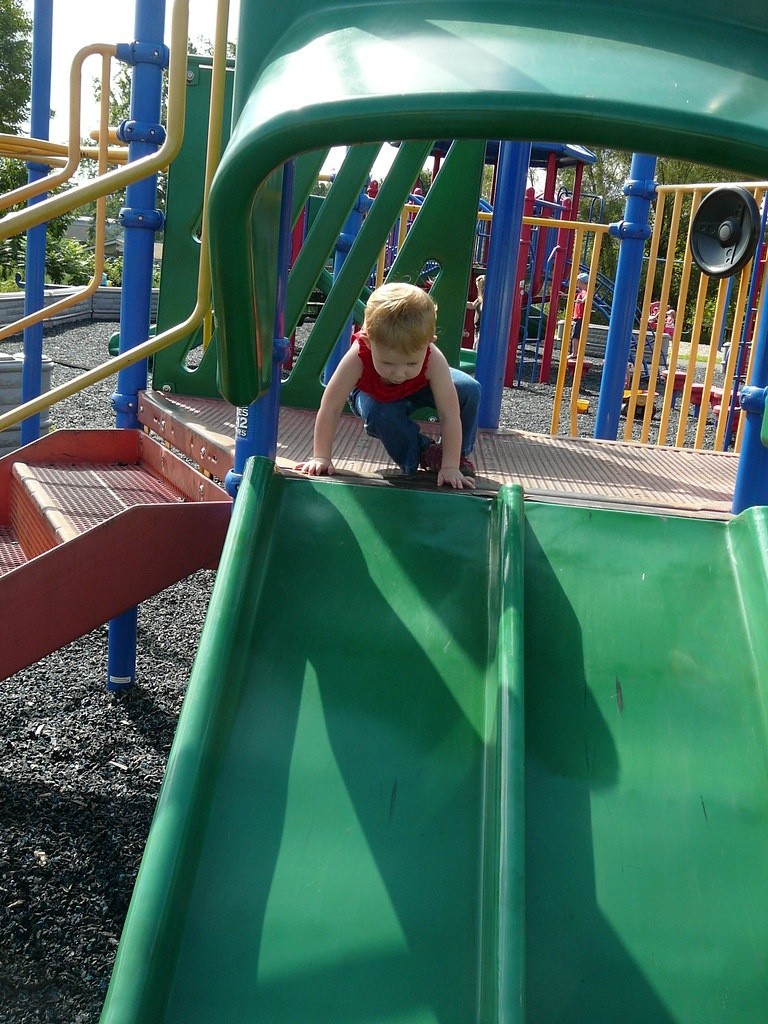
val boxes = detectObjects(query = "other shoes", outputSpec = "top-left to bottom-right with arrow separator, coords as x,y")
567,355 -> 577,360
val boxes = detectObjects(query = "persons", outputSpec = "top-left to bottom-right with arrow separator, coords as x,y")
467,275 -> 485,352
648,298 -> 675,344
558,274 -> 589,358
293,283 -> 481,489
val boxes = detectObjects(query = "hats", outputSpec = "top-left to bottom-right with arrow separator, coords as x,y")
576,273 -> 589,284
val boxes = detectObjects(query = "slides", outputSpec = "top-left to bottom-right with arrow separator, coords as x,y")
95,455 -> 768,1024
315,267 -> 478,375
107,313 -> 216,360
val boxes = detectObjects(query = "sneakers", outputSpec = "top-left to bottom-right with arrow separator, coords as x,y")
459,457 -> 476,478
419,442 -> 442,473
